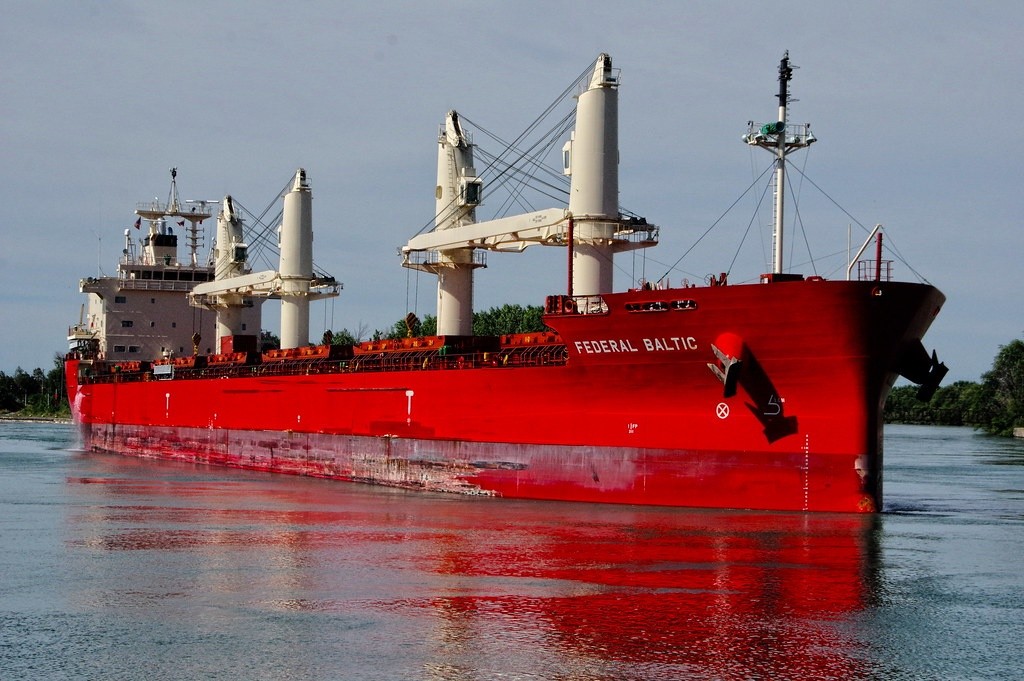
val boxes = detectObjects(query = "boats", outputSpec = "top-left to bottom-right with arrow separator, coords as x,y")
63,49 -> 949,516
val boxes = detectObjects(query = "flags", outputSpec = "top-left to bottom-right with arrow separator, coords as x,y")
177,220 -> 185,226
134,217 -> 142,230
